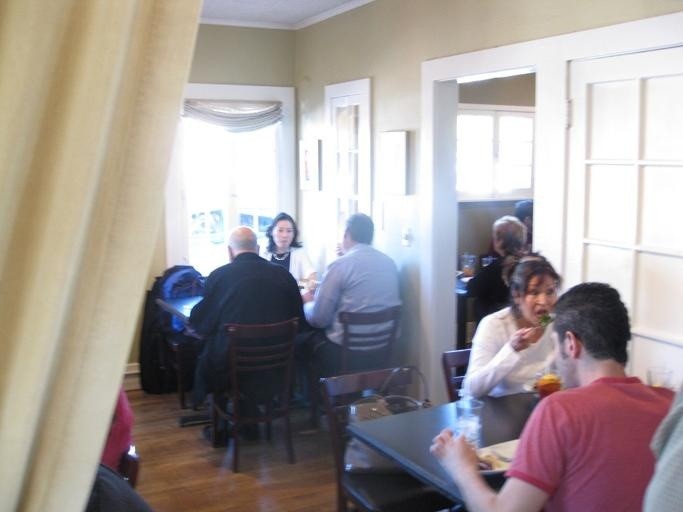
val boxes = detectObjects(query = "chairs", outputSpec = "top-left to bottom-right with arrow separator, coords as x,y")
442,348 -> 471,401
319,368 -> 462,511
300,306 -> 403,437
217,317 -> 300,474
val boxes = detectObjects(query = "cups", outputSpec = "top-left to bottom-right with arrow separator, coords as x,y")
455,399 -> 484,441
462,254 -> 476,277
537,383 -> 560,399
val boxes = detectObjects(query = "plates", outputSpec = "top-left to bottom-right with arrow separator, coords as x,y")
476,439 -> 520,476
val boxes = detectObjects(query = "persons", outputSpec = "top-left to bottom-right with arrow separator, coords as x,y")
464,214 -> 530,329
257,212 -> 303,286
187,226 -> 304,448
286,210 -> 402,426
486,198 -> 534,257
458,251 -> 564,401
426,279 -> 681,512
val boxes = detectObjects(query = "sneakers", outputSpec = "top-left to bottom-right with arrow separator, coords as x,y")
204,425 -> 226,446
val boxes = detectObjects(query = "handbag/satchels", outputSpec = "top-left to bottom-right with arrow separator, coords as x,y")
343,368 -> 429,474
155,266 -> 203,335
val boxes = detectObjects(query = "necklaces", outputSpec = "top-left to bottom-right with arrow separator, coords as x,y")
270,250 -> 290,262
